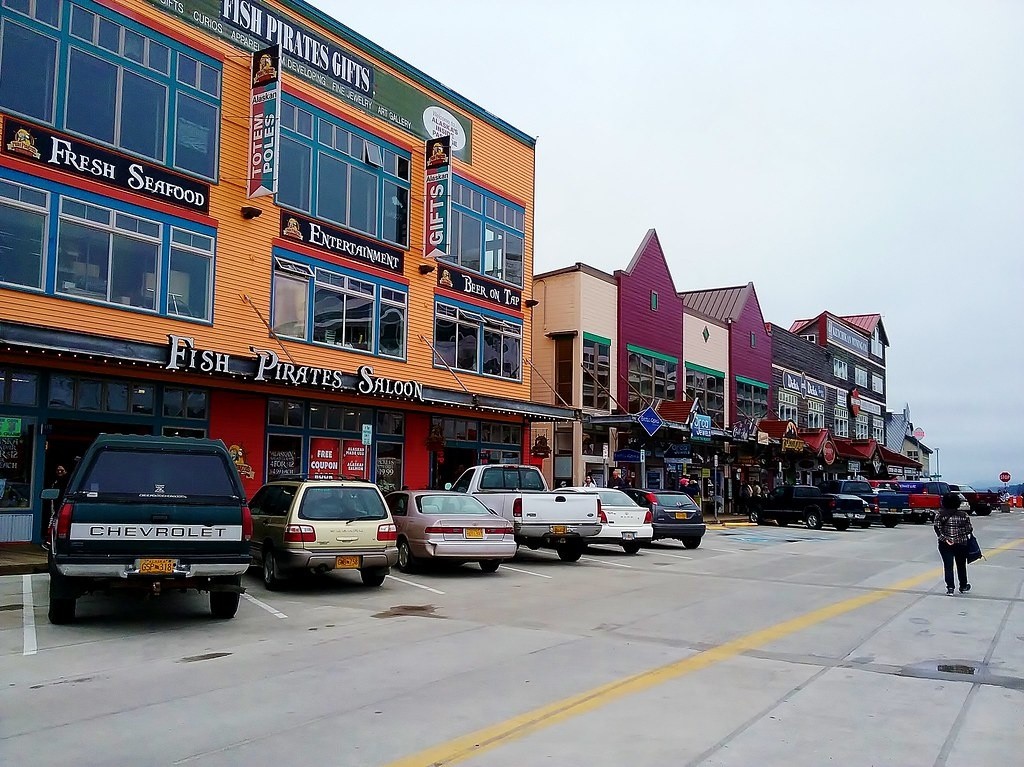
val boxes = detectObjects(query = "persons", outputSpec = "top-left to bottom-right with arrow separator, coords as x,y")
934,494 -> 973,595
987,489 -> 1024,513
738,480 -> 788,515
397,486 -> 409,513
677,478 -> 700,498
583,475 -> 595,488
607,471 -> 624,489
40,464 -> 70,550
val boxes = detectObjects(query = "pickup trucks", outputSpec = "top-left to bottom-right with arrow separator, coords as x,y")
949,485 -> 998,516
749,484 -> 865,530
449,464 -> 603,562
867,480 -> 940,524
817,479 -> 912,528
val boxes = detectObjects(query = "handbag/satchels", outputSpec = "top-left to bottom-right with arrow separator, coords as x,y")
966,532 -> 982,564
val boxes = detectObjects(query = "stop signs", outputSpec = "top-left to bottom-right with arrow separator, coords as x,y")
1000,472 -> 1010,482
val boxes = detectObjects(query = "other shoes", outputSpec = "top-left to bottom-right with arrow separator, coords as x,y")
959,584 -> 971,593
946,589 -> 953,596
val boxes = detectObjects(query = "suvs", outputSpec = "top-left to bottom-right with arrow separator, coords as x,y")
249,474 -> 400,592
900,481 -> 970,521
39,433 -> 255,624
619,488 -> 707,549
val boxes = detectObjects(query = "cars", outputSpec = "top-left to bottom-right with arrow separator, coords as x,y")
550,487 -> 653,555
383,489 -> 517,572
